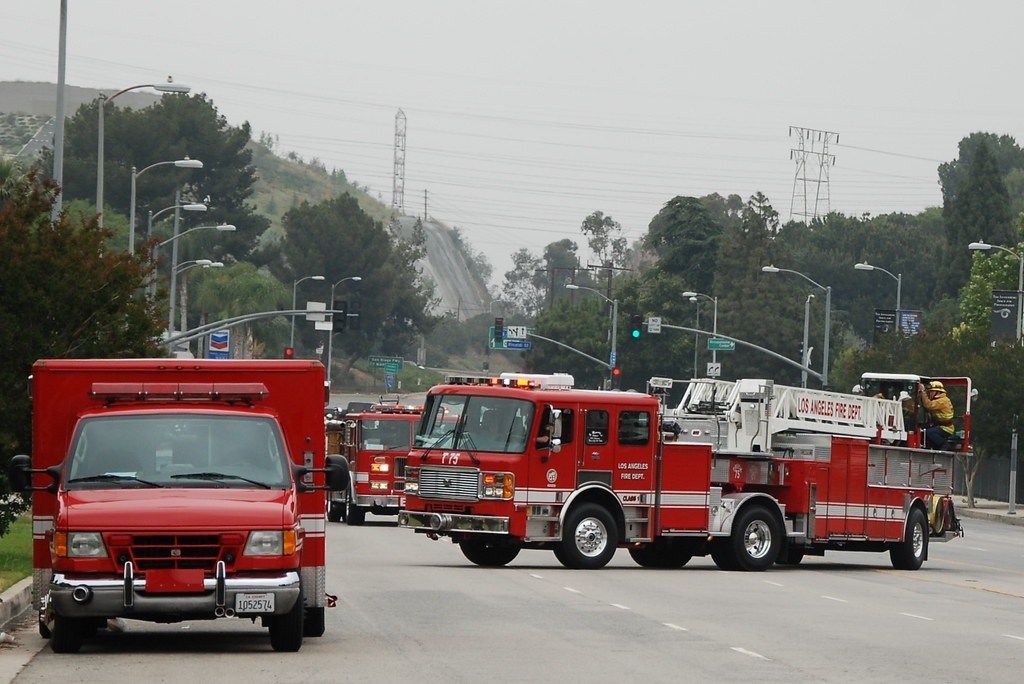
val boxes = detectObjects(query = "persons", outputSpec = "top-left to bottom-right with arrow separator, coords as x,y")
917,381 -> 954,450
535,427 -> 552,449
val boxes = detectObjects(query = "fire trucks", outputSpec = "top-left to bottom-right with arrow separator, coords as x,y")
8,355 -> 354,655
395,369 -> 980,569
324,398 -> 461,526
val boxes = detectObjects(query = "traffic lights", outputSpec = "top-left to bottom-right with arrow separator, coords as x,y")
494,317 -> 504,343
284,347 -> 293,359
612,366 -> 621,388
630,311 -> 642,340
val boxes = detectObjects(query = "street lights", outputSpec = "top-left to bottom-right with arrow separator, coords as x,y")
761,266 -> 832,390
124,159 -> 204,335
144,202 -> 207,320
854,262 -> 902,339
166,258 -> 225,358
968,241 -> 1024,515
151,222 -> 238,336
565,284 -> 619,389
94,76 -> 194,262
289,275 -> 326,349
326,276 -> 363,380
682,291 -> 718,379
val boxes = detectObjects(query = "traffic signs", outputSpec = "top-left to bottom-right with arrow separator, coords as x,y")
708,337 -> 736,351
488,325 -> 537,351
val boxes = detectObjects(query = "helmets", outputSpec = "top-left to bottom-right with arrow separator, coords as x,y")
927,381 -> 946,392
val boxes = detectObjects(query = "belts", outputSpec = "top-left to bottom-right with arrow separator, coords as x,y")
934,420 -> 954,426
